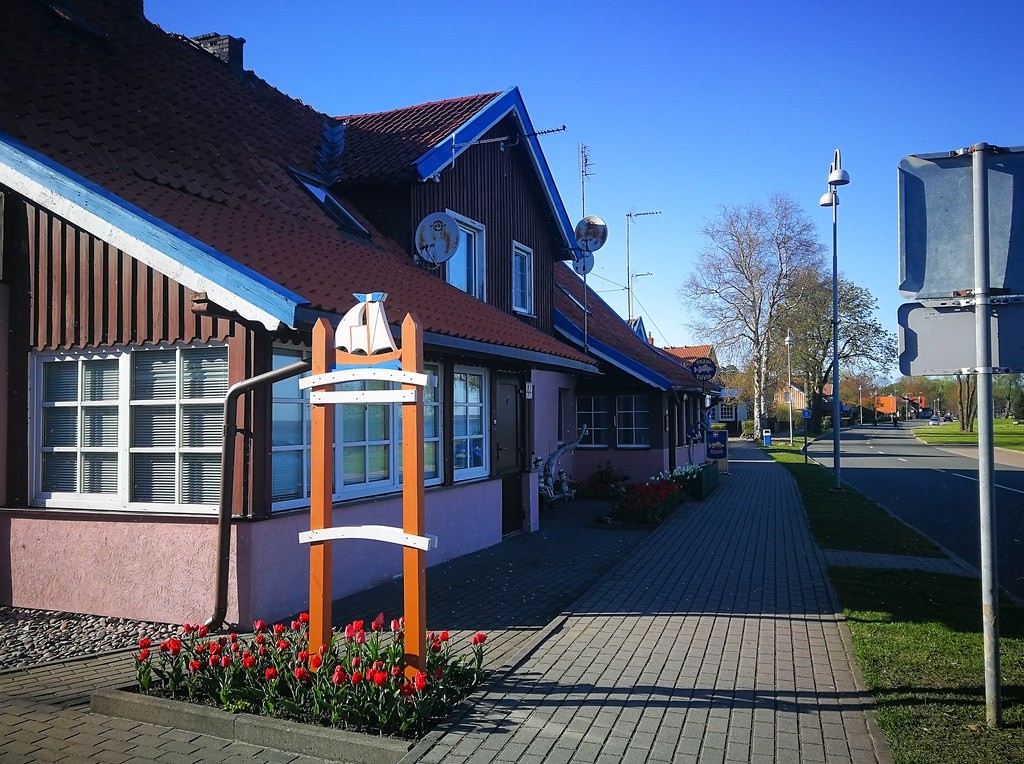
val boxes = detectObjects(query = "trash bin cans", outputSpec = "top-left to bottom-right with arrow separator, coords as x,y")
763,429 -> 772,446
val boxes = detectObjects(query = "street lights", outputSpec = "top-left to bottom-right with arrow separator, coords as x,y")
937,398 -> 940,416
858,385 -> 864,424
905,396 -> 909,421
933,399 -> 936,417
785,326 -> 796,446
818,148 -> 850,492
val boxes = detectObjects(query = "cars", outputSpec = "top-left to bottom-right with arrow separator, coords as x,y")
928,417 -> 940,426
944,415 -> 953,422
